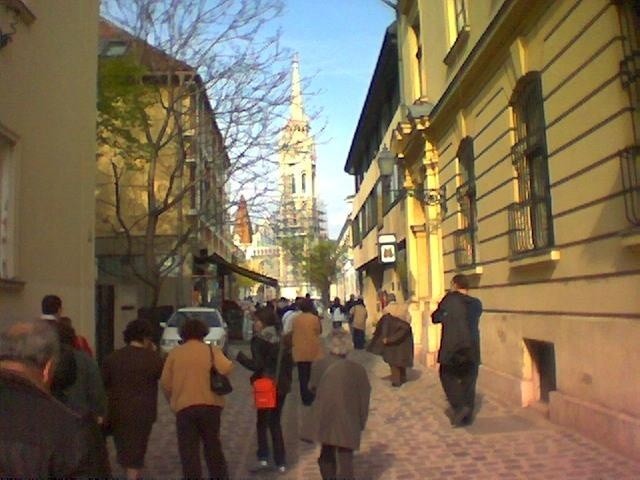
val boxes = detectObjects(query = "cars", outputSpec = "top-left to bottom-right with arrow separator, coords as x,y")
158,305 -> 230,357
308,295 -> 335,303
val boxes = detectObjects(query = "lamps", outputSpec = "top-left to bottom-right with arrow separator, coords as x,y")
378,142 -> 447,208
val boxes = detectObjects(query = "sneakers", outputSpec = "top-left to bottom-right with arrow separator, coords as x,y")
452,405 -> 475,427
249,460 -> 286,474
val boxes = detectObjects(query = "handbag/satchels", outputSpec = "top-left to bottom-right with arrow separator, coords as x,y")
253,377 -> 277,408
210,367 -> 232,395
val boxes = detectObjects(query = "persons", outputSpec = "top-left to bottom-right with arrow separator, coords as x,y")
430,273 -> 483,427
40,291 -> 65,324
252,291 -> 368,364
288,298 -> 327,407
0,316 -> 114,479
307,326 -> 372,479
51,319 -> 108,430
160,315 -> 234,479
369,293 -> 414,387
100,317 -> 164,479
52,315 -> 95,358
236,306 -> 295,476
